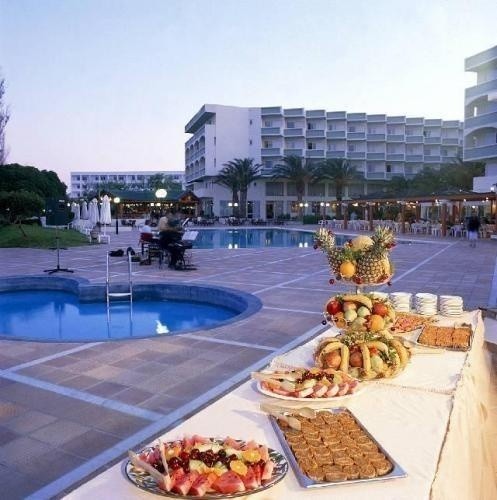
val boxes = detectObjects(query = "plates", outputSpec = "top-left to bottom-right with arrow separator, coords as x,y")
256,369 -> 362,409
123,435 -> 289,500
370,291 -> 464,318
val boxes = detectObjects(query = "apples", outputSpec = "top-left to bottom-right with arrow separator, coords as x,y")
343,301 -> 357,312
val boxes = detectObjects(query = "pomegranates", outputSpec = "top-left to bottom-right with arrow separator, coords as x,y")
372,303 -> 388,316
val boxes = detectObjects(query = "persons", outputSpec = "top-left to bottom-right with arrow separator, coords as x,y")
156,211 -> 175,234
479,217 -> 496,224
118,203 -> 195,226
159,218 -> 184,270
142,219 -> 152,233
466,211 -> 481,248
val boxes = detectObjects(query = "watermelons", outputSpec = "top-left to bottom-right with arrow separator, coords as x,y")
157,434 -> 274,496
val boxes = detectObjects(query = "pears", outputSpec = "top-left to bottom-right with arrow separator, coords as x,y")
345,308 -> 357,323
357,306 -> 370,319
355,317 -> 366,326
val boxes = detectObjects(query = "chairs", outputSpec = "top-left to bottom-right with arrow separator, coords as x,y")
148,244 -> 185,269
191,216 -> 284,226
318,219 -> 497,239
67,219 -> 110,244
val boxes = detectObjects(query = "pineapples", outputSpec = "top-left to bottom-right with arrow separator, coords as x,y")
356,224 -> 392,284
314,228 -> 348,275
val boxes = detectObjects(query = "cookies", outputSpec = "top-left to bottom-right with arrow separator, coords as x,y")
279,410 -> 393,482
418,325 -> 470,348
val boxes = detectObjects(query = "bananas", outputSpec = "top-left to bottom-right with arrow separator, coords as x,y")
315,330 -> 408,373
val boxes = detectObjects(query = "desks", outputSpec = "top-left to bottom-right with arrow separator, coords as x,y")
141,242 -> 160,257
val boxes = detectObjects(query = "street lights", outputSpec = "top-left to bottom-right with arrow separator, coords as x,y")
226,229 -> 239,249
297,232 -> 309,248
113,196 -> 121,235
319,201 -> 330,227
227,201 -> 239,225
489,183 -> 496,220
155,189 -> 167,218
300,201 -> 309,225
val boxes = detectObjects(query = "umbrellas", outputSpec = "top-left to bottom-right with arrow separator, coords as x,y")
80,200 -> 87,221
99,194 -> 112,235
70,202 -> 76,219
88,197 -> 100,226
85,201 -> 92,220
74,203 -> 80,220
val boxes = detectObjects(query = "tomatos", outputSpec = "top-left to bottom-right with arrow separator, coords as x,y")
327,300 -> 341,315
